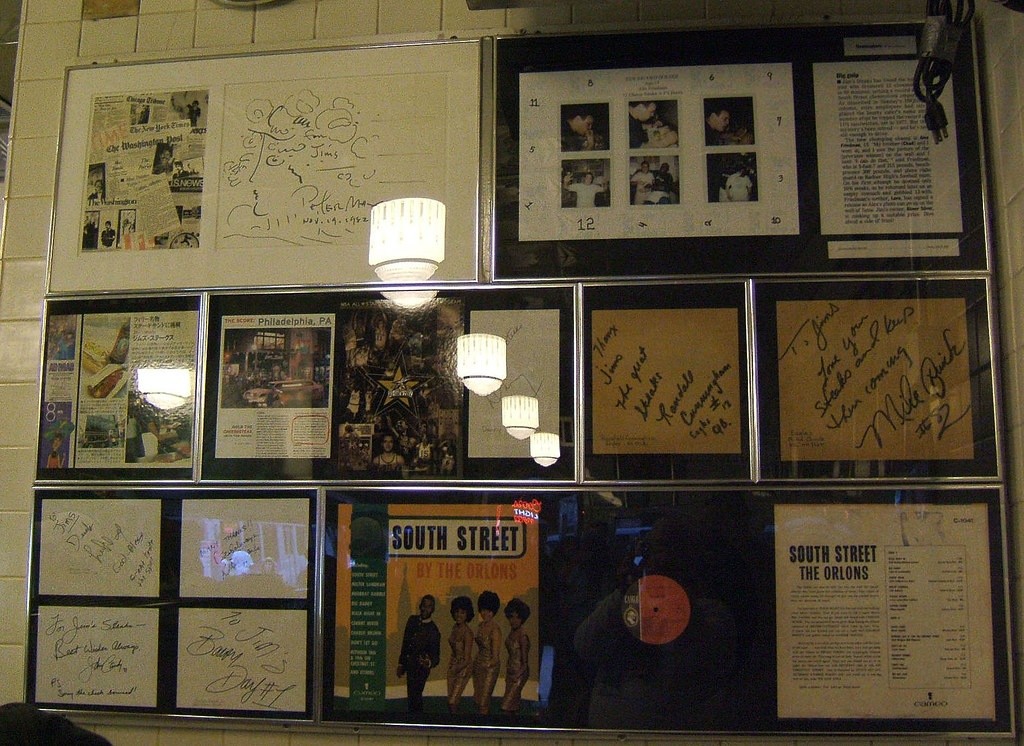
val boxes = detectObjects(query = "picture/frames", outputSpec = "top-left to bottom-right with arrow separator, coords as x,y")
24,15 -> 1016,743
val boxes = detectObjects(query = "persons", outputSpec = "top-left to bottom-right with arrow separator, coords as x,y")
474,590 -> 503,716
173,162 -> 189,178
560,105 -> 596,152
101,221 -> 115,249
446,596 -> 475,717
342,304 -> 459,478
396,594 -> 441,722
502,598 -> 532,713
627,100 -> 678,149
706,154 -> 753,202
187,100 -> 201,126
704,103 -> 753,147
630,160 -> 673,204
564,171 -> 607,208
88,180 -> 105,206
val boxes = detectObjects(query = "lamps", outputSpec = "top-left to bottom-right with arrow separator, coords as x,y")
530,433 -> 561,468
500,395 -> 540,440
134,366 -> 194,413
368,197 -> 508,397
